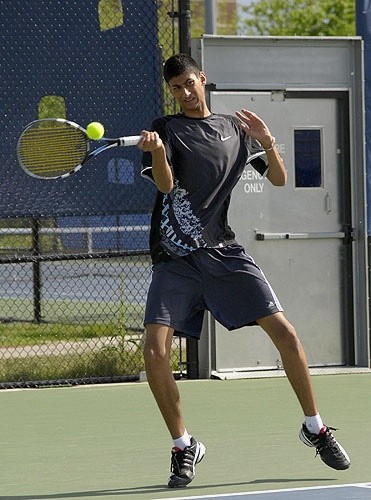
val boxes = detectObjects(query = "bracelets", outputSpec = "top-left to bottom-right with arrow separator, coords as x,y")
263,138 -> 276,153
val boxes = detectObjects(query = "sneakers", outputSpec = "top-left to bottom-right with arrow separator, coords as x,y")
168,437 -> 206,487
300,422 -> 351,470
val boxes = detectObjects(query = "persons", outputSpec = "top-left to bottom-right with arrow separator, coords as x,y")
137,54 -> 351,487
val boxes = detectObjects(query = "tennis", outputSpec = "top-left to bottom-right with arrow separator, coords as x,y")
86,121 -> 105,140
